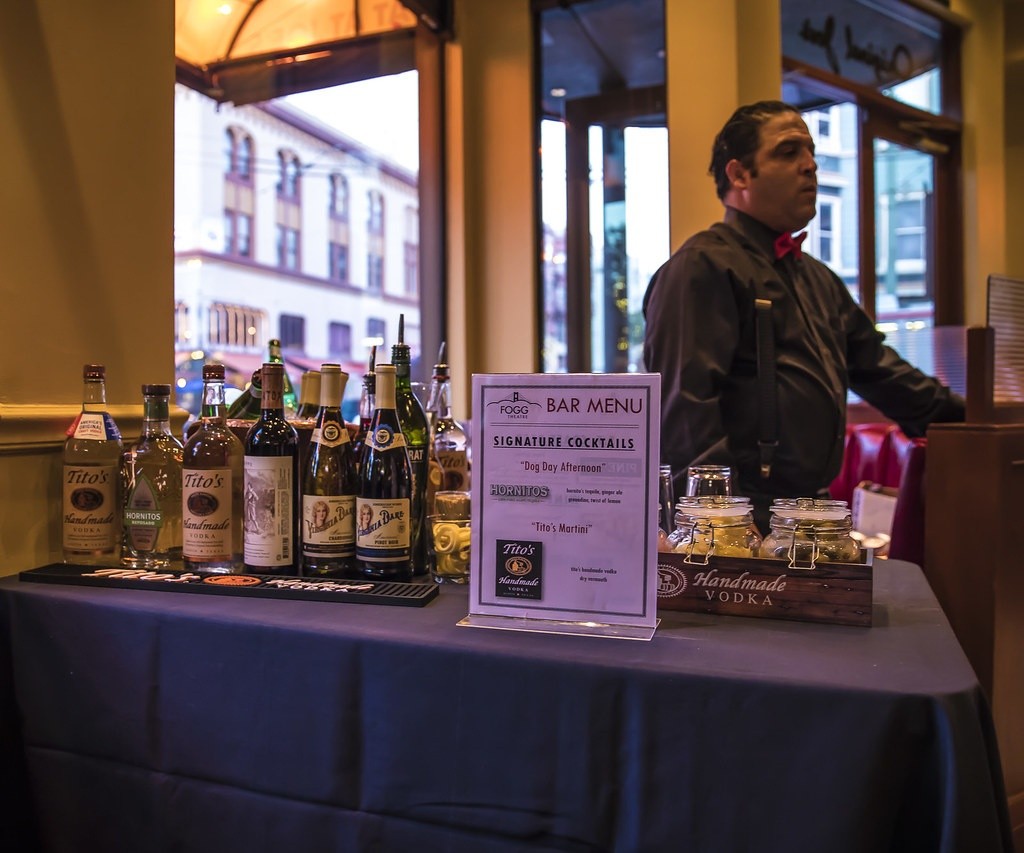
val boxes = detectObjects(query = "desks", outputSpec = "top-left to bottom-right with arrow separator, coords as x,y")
0,553 -> 1020,853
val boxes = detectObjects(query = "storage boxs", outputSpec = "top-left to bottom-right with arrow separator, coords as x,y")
657,540 -> 874,626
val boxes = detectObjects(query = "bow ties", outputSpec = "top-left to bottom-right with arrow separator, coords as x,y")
774,231 -> 807,260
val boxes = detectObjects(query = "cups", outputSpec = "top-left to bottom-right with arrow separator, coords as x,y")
684,465 -> 732,497
656,465 -> 675,535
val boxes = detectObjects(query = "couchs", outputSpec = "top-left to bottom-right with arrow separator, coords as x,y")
828,419 -> 931,558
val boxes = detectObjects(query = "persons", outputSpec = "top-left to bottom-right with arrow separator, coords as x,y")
640,102 -> 966,541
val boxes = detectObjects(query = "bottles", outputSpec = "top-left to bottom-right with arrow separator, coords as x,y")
761,495 -> 861,575
119,386 -> 185,570
667,495 -> 760,567
181,365 -> 244,574
62,363 -> 123,567
232,313 -> 472,585
